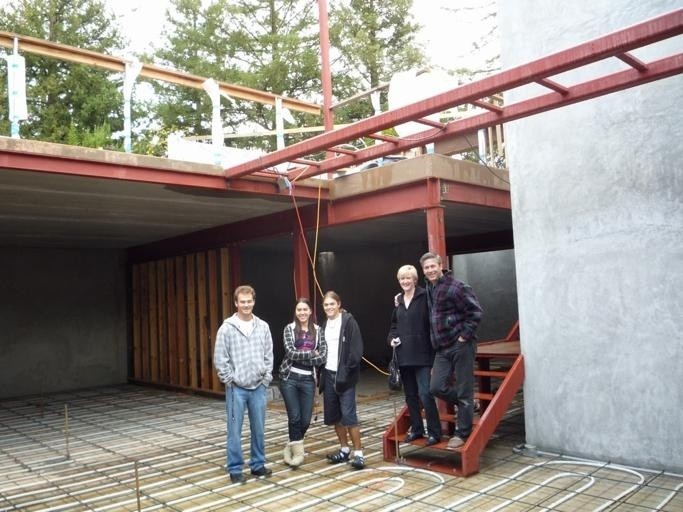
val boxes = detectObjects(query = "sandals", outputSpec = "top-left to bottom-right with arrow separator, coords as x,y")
326,450 -> 350,463
351,455 -> 365,467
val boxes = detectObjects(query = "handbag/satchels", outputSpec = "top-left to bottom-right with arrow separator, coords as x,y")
389,367 -> 403,393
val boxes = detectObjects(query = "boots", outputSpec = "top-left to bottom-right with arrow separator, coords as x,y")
283,438 -> 305,467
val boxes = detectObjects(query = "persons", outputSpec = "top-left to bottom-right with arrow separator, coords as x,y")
387,264 -> 442,445
274,297 -> 327,468
418,252 -> 483,449
317,289 -> 366,468
212,284 -> 275,483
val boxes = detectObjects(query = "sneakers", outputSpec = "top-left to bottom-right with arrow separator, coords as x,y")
230,473 -> 246,483
405,431 -> 416,444
424,437 -> 438,447
473,401 -> 480,412
251,466 -> 272,476
445,436 -> 464,449
